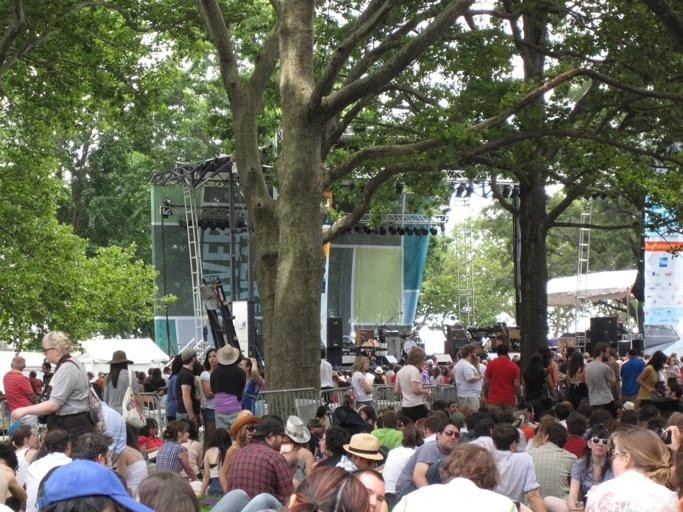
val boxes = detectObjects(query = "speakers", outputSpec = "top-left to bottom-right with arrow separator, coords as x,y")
341,356 -> 356,365
486,352 -> 498,360
591,318 -> 617,342
508,353 -> 521,361
435,354 -> 453,366
383,355 -> 399,366
327,318 -> 343,348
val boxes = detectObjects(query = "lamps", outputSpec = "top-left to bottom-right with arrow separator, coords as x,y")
330,212 -> 438,238
196,211 -> 250,234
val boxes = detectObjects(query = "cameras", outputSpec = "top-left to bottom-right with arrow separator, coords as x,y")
656,427 -> 671,443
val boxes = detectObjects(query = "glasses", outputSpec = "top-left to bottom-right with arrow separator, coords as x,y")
592,438 -> 609,446
444,431 -> 460,439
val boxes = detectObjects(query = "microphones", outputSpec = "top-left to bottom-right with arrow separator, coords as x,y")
396,312 -> 402,314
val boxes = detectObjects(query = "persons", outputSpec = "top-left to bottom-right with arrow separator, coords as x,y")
1,327 -> 682,512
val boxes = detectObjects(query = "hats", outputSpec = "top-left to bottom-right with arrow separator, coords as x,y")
230,410 -> 311,444
35,460 -> 155,512
108,351 -> 134,364
216,344 -> 239,365
374,367 -> 383,373
344,433 -> 384,460
181,349 -> 196,361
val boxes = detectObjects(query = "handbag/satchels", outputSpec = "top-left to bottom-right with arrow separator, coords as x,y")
101,400 -> 127,454
123,385 -> 147,429
